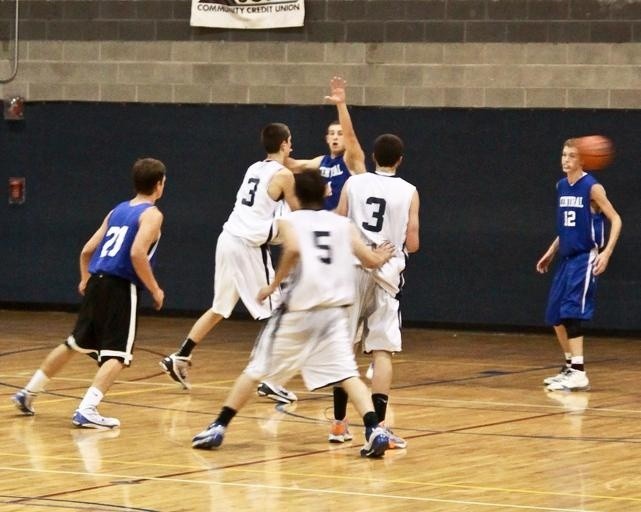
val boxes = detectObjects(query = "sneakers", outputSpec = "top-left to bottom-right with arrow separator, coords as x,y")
11,388 -> 36,415
191,422 -> 226,449
158,395 -> 192,439
328,418 -> 354,444
258,381 -> 297,405
159,352 -> 194,390
72,406 -> 122,431
256,404 -> 296,436
332,446 -> 407,488
543,365 -> 590,392
359,424 -> 408,457
72,429 -> 120,454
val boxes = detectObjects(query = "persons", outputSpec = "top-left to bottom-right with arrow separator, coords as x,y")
158,122 -> 301,406
328,132 -> 422,452
282,74 -> 368,214
533,134 -> 623,393
190,168 -> 390,458
10,156 -> 169,430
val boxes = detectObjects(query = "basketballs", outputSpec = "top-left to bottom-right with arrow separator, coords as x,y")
577,136 -> 613,171
